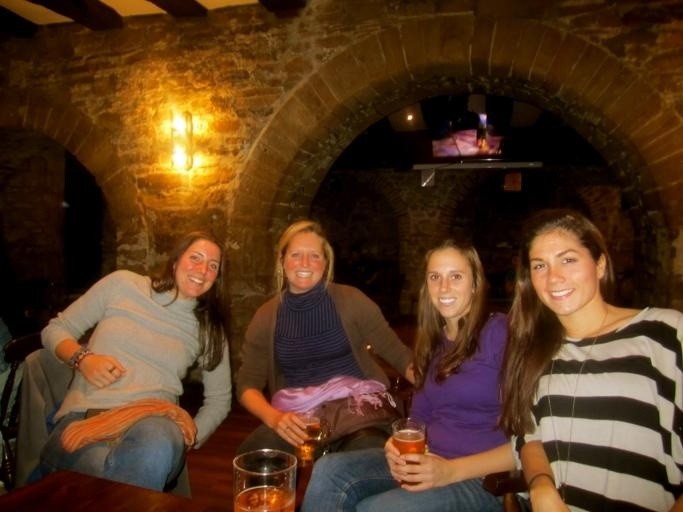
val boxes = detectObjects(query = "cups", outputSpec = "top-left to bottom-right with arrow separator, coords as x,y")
291,413 -> 332,468
391,418 -> 428,487
230,448 -> 298,512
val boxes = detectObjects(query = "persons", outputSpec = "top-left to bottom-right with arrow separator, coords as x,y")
293,237 -> 513,511
489,207 -> 683,511
228,219 -> 420,482
36,228 -> 236,493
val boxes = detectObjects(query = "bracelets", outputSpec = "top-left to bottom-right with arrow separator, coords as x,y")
69,348 -> 93,369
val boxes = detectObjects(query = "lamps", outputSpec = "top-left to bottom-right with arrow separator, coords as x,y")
169,111 -> 194,172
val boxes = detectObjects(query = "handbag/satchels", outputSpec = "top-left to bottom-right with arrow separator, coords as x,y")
315,393 -> 402,445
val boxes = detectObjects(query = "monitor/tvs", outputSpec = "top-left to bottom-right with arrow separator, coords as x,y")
427,110 -> 506,161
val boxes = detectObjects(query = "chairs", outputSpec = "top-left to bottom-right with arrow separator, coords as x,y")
293,354 -> 415,512
482,470 -> 528,512
0,336 -> 87,492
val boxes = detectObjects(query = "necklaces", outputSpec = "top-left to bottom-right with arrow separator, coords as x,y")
544,304 -> 612,508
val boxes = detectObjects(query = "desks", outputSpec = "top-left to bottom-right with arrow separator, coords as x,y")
0,469 -> 233,512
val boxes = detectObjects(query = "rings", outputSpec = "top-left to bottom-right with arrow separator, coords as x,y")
284,425 -> 288,432
108,366 -> 115,373
